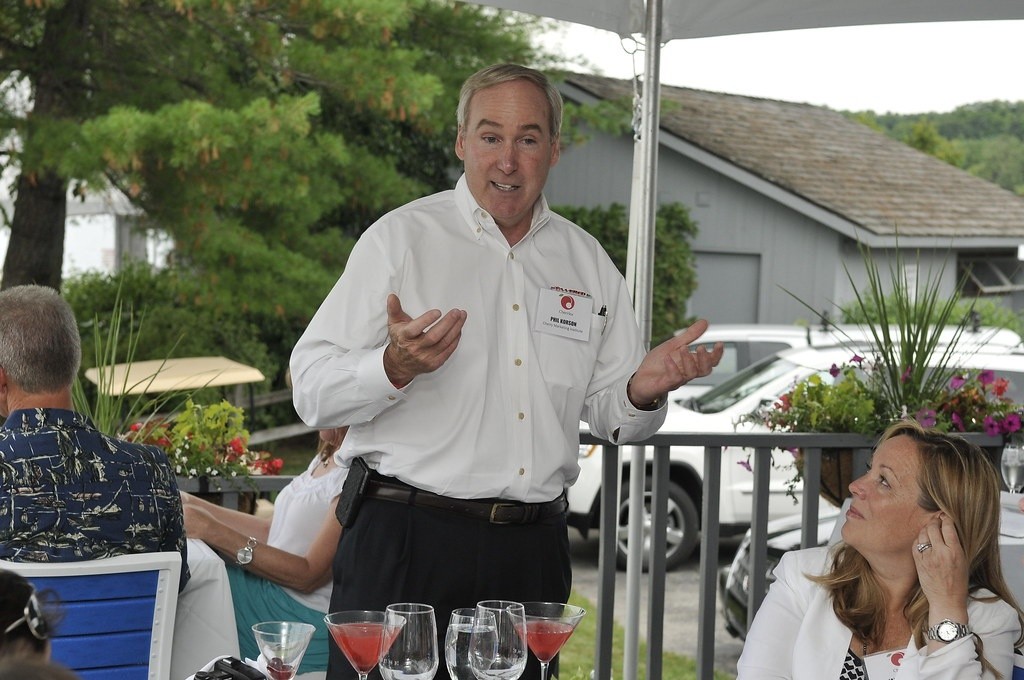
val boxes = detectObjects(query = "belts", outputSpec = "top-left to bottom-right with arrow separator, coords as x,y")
361,480 -> 569,525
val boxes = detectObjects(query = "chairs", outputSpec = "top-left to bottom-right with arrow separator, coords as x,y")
0,551 -> 182,679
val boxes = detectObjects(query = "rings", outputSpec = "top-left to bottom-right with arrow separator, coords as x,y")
917,543 -> 932,553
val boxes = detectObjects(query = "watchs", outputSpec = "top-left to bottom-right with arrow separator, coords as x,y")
927,619 -> 969,644
233,536 -> 257,568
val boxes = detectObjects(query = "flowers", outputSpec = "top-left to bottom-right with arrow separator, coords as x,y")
730,220 -> 1022,505
129,393 -> 282,508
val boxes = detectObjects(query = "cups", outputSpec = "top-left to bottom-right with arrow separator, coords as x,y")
445,608 -> 498,680
379,604 -> 439,680
251,621 -> 316,680
469,600 -> 527,680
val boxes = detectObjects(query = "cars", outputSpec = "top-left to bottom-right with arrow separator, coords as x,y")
611,345 -> 1024,642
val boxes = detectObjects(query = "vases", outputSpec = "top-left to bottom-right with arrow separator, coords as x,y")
788,446 -> 874,508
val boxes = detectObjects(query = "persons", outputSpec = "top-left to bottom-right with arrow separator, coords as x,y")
179,425 -> 349,676
0,284 -> 192,592
735,421 -> 1024,680
288,64 -> 725,680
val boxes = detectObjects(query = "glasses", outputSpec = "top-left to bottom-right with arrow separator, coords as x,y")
4,583 -> 51,641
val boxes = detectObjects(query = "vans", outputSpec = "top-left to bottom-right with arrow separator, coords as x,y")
569,324 -> 1022,543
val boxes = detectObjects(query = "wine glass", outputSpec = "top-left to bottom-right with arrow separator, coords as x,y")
324,611 -> 407,680
1001,442 -> 1024,494
507,602 -> 586,680
